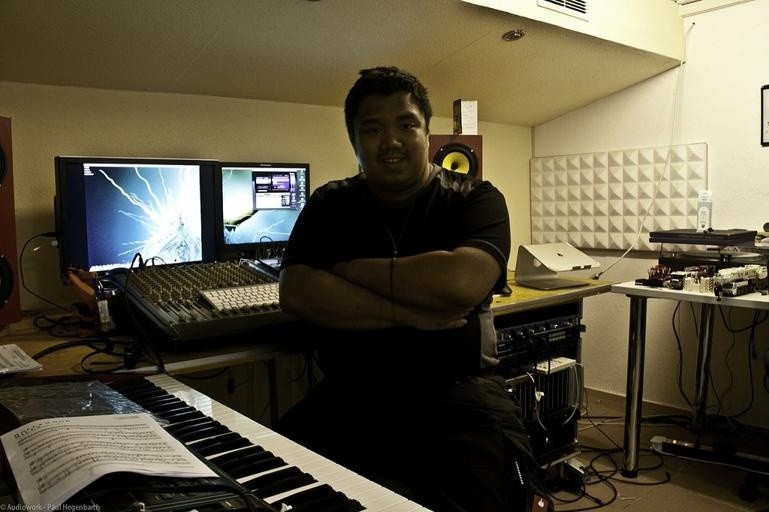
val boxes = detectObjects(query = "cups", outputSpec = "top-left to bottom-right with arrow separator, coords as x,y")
96,288 -> 122,335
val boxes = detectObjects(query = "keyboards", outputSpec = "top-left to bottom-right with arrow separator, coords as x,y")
199,282 -> 279,312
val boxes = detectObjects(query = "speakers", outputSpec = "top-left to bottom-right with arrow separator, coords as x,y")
0,115 -> 21,327
429,135 -> 482,181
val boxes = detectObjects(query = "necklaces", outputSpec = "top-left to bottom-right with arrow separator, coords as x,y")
374,190 -> 418,256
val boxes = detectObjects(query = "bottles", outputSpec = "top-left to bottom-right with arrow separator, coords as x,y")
697,189 -> 712,234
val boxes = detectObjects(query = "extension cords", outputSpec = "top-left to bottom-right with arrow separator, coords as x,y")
649,435 -> 769,474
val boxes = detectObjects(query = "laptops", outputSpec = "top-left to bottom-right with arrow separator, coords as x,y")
649,228 -> 757,245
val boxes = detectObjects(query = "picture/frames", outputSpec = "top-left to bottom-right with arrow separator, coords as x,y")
761,85 -> 769,147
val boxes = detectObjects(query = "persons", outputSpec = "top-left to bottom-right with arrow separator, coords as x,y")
269,65 -> 537,512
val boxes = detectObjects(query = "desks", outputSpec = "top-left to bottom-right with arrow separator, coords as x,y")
0,270 -> 768,509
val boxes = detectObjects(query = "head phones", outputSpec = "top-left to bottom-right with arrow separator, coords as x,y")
81,337 -> 137,374
89,278 -> 121,340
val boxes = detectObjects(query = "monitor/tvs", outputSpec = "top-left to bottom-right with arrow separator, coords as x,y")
55,156 -> 224,285
221,162 -> 310,261
